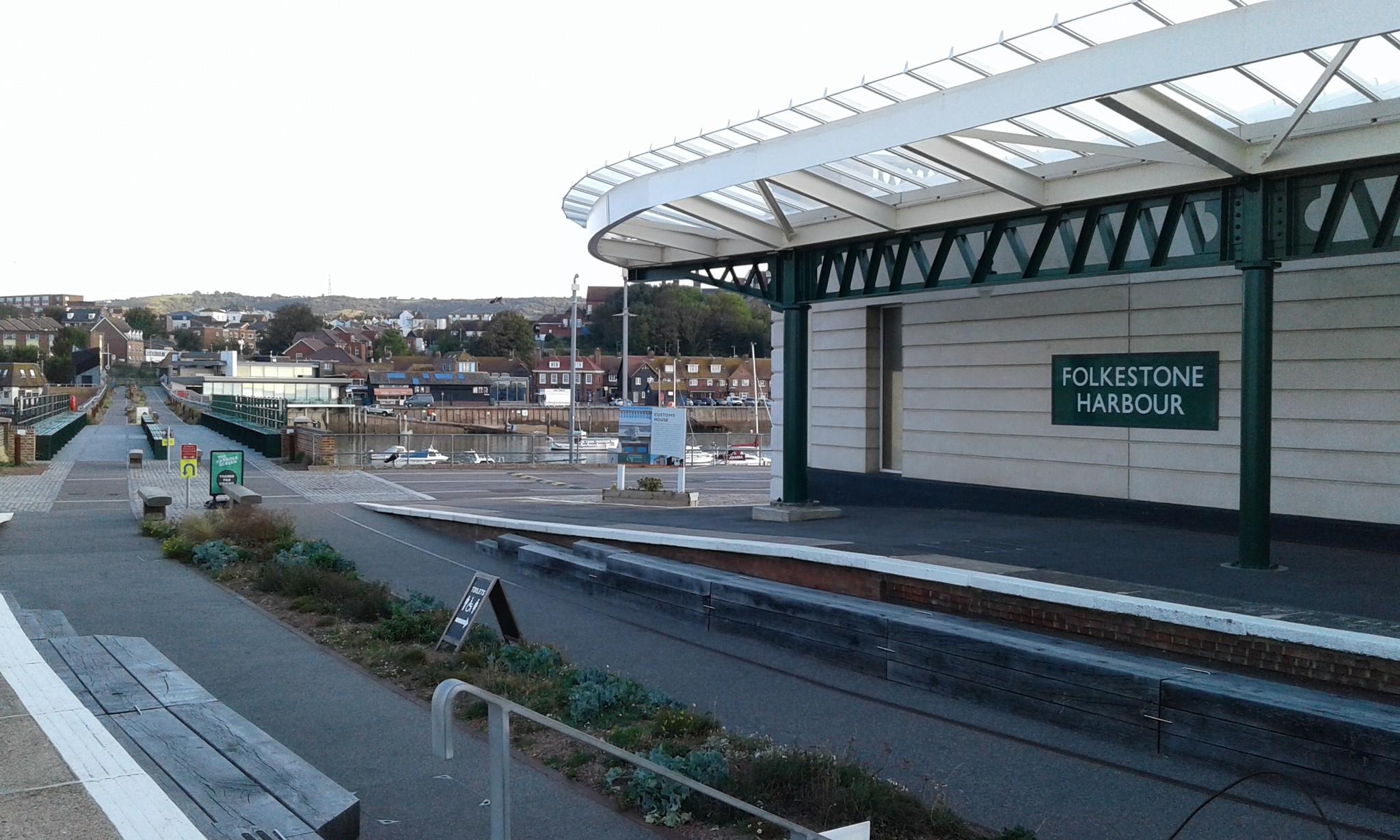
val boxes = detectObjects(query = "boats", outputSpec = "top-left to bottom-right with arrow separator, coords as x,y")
712,434 -> 772,467
550,414 -> 622,450
370,445 -> 496,470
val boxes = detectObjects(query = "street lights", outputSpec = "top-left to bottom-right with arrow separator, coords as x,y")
150,334 -> 156,368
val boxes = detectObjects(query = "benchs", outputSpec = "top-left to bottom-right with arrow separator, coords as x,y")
197,448 -> 203,467
138,486 -> 172,522
222,483 -> 262,510
129,449 -> 143,469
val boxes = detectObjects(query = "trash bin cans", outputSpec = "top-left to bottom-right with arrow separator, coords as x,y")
489,394 -> 495,406
361,398 -> 371,405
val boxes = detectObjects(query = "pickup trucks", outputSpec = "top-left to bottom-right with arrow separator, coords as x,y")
360,403 -> 394,417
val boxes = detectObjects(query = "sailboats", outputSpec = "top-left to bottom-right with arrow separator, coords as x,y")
668,358 -> 721,465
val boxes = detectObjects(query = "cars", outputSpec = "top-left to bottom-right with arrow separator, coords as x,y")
609,398 -> 633,407
684,395 -> 773,407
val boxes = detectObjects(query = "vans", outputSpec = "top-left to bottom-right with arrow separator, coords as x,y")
403,394 -> 434,408
